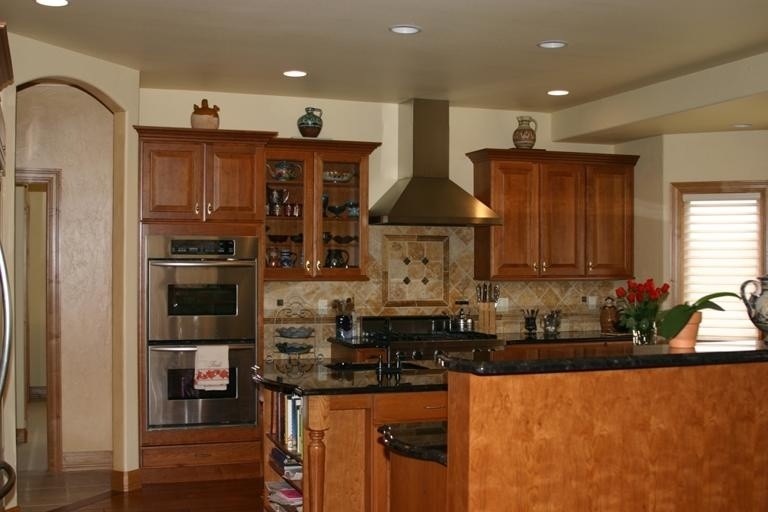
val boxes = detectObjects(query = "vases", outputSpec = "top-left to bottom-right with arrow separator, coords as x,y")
631,321 -> 659,344
513,116 -> 537,148
297,108 -> 323,135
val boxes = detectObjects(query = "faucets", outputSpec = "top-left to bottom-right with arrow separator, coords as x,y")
386,372 -> 391,386
386,345 -> 391,369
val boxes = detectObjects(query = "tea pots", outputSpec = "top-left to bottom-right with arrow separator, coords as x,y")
265,159 -> 303,181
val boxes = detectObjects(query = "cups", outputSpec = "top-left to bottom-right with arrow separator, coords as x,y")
284,202 -> 294,216
294,204 -> 303,217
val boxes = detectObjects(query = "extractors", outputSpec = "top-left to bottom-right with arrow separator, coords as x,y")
366,98 -> 503,228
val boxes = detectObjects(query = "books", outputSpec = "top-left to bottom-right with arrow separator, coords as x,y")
263,391 -> 304,512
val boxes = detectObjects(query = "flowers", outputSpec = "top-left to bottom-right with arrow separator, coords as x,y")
615,278 -> 671,320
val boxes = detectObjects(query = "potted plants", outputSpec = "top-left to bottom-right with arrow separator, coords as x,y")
662,292 -> 741,349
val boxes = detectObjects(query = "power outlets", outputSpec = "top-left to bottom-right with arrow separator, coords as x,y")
587,296 -> 598,306
496,298 -> 509,311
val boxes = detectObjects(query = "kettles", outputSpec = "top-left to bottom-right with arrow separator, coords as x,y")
442,300 -> 473,333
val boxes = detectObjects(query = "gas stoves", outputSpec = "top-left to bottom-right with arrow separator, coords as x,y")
362,331 -> 496,361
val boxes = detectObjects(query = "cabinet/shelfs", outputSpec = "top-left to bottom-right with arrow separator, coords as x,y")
133,124 -> 279,235
465,145 -> 641,282
377,391 -> 448,512
264,139 -> 382,281
255,384 -> 376,511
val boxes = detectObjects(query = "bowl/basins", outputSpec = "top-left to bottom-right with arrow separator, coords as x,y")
267,232 -> 331,244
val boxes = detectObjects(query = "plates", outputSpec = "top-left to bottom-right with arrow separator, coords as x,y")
275,342 -> 314,354
274,327 -> 317,338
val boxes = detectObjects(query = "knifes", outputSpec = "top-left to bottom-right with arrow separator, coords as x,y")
475,282 -> 501,303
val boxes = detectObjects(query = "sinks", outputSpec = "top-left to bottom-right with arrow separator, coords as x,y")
323,362 -> 431,371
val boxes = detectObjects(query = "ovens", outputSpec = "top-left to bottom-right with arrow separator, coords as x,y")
145,233 -> 256,429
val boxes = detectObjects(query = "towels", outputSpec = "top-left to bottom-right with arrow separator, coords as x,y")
194,345 -> 229,391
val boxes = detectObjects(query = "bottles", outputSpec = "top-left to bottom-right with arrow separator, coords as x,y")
267,186 -> 289,217
741,273 -> 768,346
321,192 -> 329,217
599,296 -> 624,333
